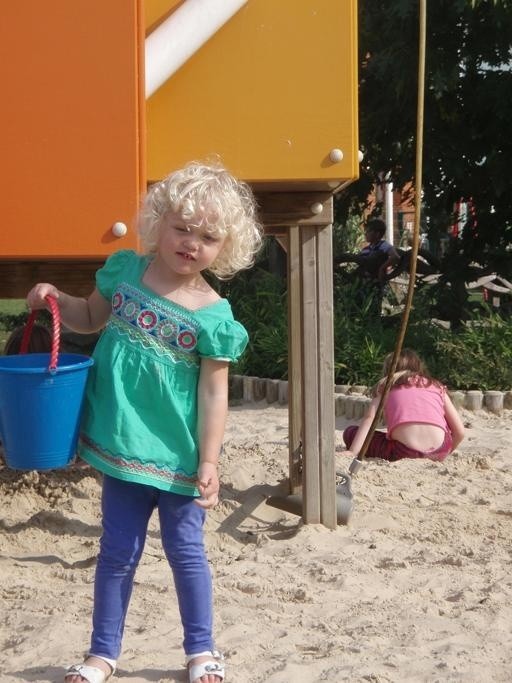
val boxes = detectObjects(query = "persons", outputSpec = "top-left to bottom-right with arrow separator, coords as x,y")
28,166 -> 262,683
351,220 -> 400,316
337,349 -> 464,462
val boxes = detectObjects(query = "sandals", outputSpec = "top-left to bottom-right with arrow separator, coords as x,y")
63,652 -> 118,683
184,650 -> 226,683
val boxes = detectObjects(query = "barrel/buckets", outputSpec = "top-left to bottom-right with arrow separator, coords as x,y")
0,295 -> 94,474
